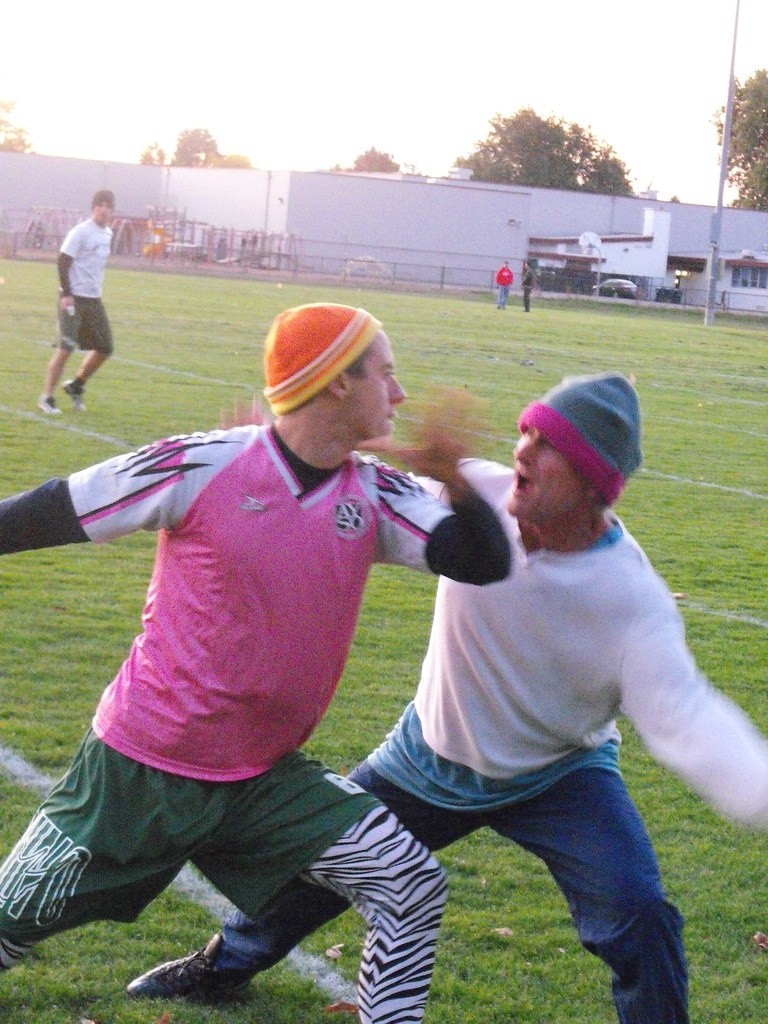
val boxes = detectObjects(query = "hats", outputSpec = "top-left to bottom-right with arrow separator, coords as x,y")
92,190 -> 115,203
519,372 -> 643,508
262,301 -> 382,416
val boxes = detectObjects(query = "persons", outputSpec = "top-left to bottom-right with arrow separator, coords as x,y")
0,302 -> 514,1024
127,371 -> 768,1024
37,189 -> 117,415
496,260 -> 533,312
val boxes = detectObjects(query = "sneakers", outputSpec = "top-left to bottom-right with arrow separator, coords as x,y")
126,934 -> 253,1003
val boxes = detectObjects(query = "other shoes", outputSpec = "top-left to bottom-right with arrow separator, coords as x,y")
37,395 -> 63,415
61,379 -> 87,412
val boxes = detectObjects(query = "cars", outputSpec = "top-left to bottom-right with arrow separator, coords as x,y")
590,278 -> 639,300
